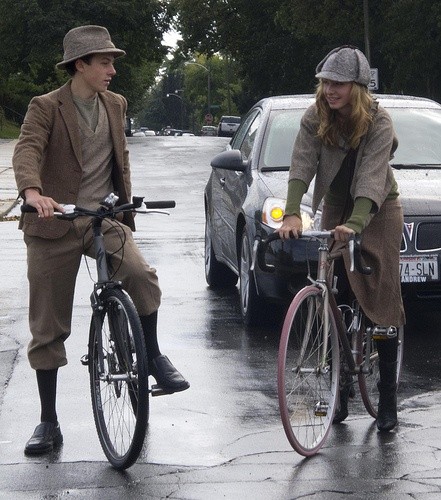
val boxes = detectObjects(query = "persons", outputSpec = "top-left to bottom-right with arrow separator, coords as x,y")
273,45 -> 406,434
12,24 -> 186,453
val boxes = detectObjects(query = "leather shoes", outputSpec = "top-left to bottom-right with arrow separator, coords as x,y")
147,354 -> 189,392
23,423 -> 65,456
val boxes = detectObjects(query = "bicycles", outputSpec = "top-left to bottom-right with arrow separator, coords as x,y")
19,194 -> 177,472
253,228 -> 404,458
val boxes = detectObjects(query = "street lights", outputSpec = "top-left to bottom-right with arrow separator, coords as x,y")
167,93 -> 183,131
184,61 -> 211,125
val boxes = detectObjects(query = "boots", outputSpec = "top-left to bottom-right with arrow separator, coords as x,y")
378,359 -> 397,431
331,376 -> 356,422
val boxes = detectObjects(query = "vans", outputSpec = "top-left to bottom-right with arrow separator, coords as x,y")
217,115 -> 242,137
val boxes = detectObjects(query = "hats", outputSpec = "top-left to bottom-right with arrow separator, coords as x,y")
57,25 -> 127,69
314,44 -> 372,87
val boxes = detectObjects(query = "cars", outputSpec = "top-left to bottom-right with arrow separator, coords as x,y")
200,125 -> 217,136
199,92 -> 441,335
130,125 -> 195,137
